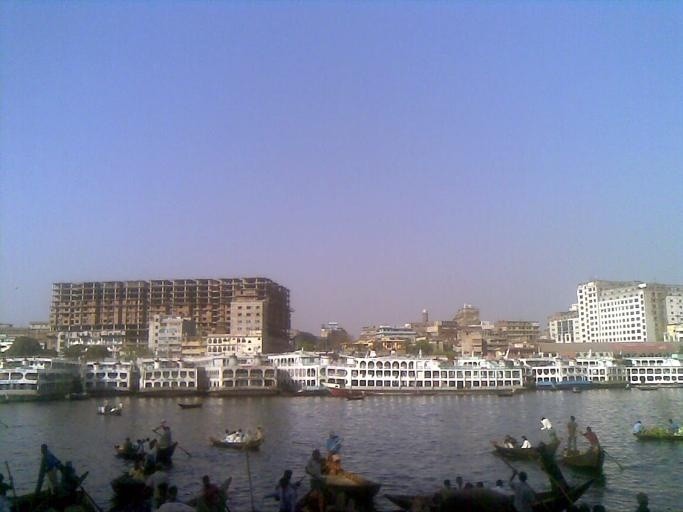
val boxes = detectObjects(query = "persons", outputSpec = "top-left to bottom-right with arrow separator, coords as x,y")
124,423 -> 197,512
273,432 -> 345,512
432,469 -> 650,512
633,420 -> 643,433
567,415 -> 601,453
668,418 -> 679,433
41,443 -> 65,497
504,418 -> 553,449
217,425 -> 262,443
0,473 -> 13,512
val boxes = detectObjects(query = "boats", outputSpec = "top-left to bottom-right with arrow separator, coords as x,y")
306,450 -> 382,500
634,429 -> 683,440
489,436 -> 607,471
384,480 -> 592,507
0,470 -> 232,511
211,438 -> 263,448
179,403 -> 205,409
497,391 -> 514,396
328,386 -> 366,398
637,385 -> 659,390
116,442 -> 178,460
97,405 -> 123,415
572,387 -> 582,393
